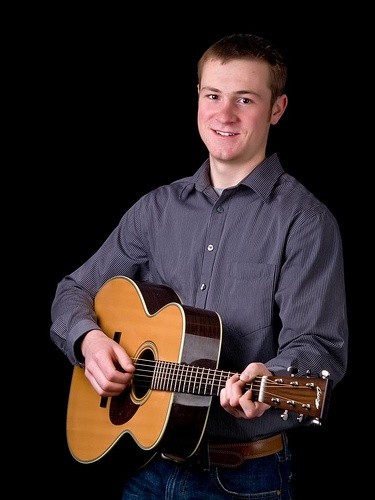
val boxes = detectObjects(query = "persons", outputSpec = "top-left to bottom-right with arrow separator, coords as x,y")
50,35 -> 350,500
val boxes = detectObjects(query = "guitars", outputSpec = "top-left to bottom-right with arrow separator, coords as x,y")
65,275 -> 334,459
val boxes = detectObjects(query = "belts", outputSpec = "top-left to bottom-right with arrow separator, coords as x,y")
196,434 -> 285,468
